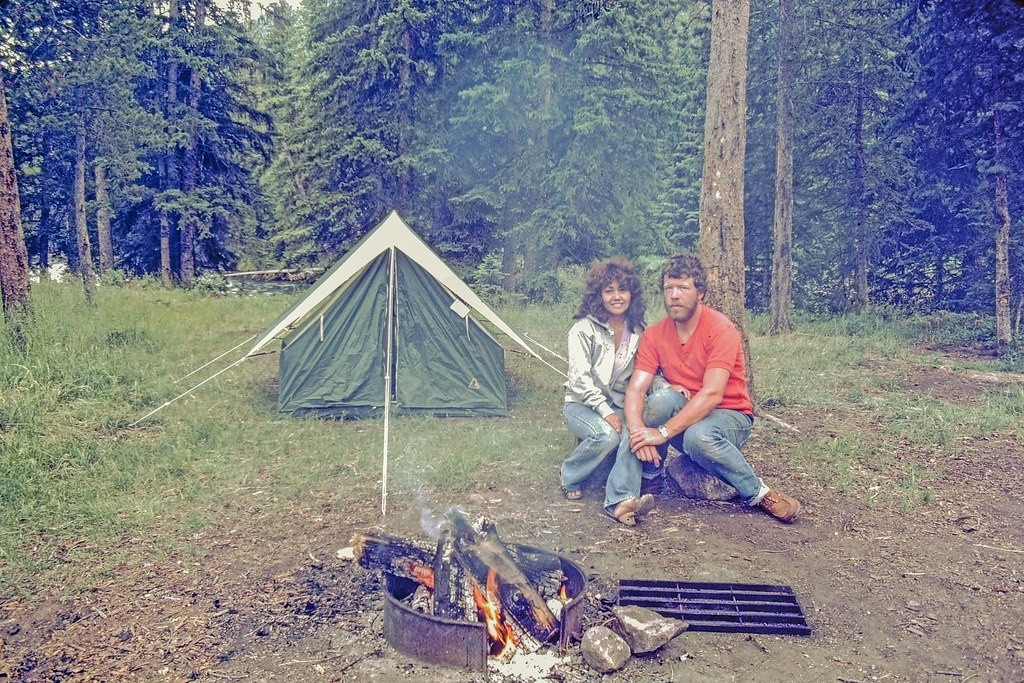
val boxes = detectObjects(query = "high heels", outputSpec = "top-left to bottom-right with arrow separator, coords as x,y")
559,472 -> 582,500
614,494 -> 655,526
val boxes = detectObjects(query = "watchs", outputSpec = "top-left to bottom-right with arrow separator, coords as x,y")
658,424 -> 670,441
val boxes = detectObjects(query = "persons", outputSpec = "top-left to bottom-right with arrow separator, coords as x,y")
558,258 -> 655,526
624,254 -> 800,521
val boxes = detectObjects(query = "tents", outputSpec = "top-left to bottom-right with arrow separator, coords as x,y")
131,210 -> 569,513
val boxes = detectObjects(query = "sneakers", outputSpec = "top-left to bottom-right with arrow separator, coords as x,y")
759,491 -> 801,521
643,475 -> 662,493
